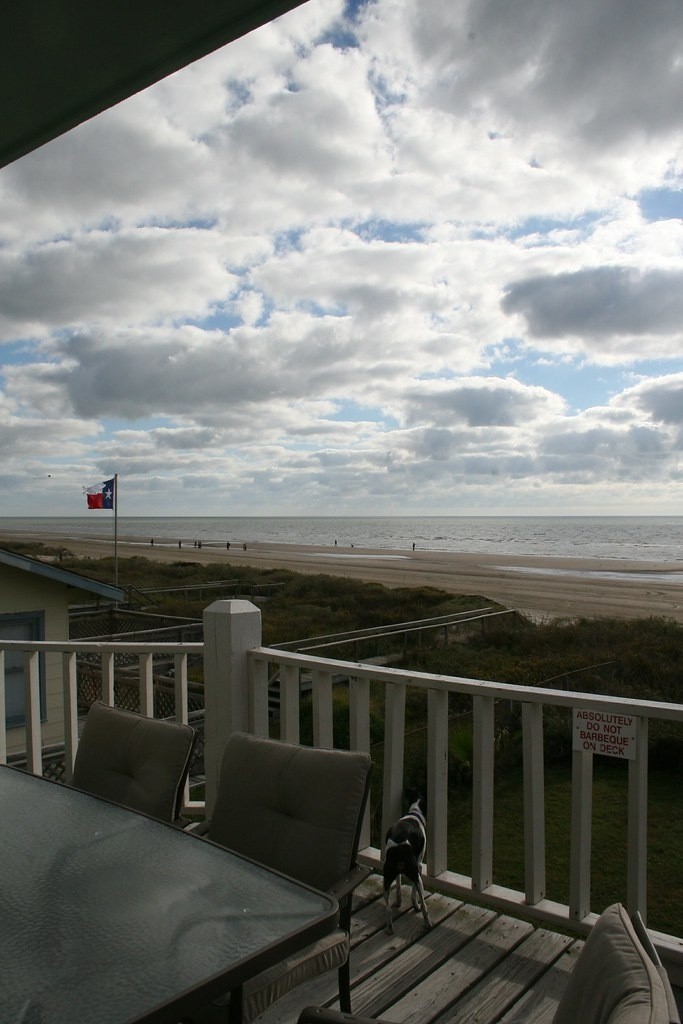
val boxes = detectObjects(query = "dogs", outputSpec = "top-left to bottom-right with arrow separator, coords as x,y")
383,781 -> 432,933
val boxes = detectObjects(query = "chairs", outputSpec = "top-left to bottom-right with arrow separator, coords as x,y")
203,732 -> 376,1024
296,903 -> 681,1023
69,701 -> 201,825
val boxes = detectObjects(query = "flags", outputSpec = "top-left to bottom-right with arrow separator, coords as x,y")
85,477 -> 115,510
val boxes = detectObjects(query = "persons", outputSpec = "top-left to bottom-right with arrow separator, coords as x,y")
178,539 -> 182,550
413,542 -> 416,552
334,539 -> 337,547
151,539 -> 153,546
193,539 -> 202,550
243,543 -> 247,552
226,541 -> 231,551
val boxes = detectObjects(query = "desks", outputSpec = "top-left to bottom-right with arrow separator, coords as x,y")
1,764 -> 341,1024
